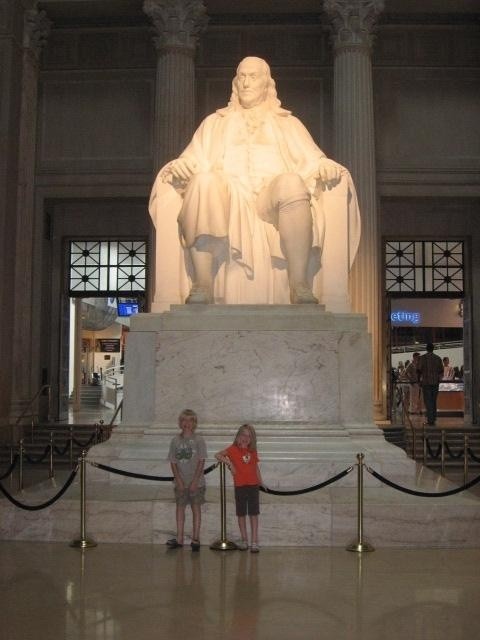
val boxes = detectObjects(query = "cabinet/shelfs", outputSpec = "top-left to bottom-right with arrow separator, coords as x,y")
437,382 -> 464,417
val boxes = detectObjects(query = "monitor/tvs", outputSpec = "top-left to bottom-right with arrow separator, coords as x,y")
116,302 -> 140,316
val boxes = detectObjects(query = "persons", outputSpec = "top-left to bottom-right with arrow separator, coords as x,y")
175,549 -> 205,640
165,409 -> 208,549
215,425 -> 263,553
232,550 -> 260,640
390,342 -> 464,426
160,56 -> 341,303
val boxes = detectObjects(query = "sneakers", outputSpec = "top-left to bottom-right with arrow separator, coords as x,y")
191,540 -> 200,548
238,542 -> 259,553
166,538 -> 183,547
